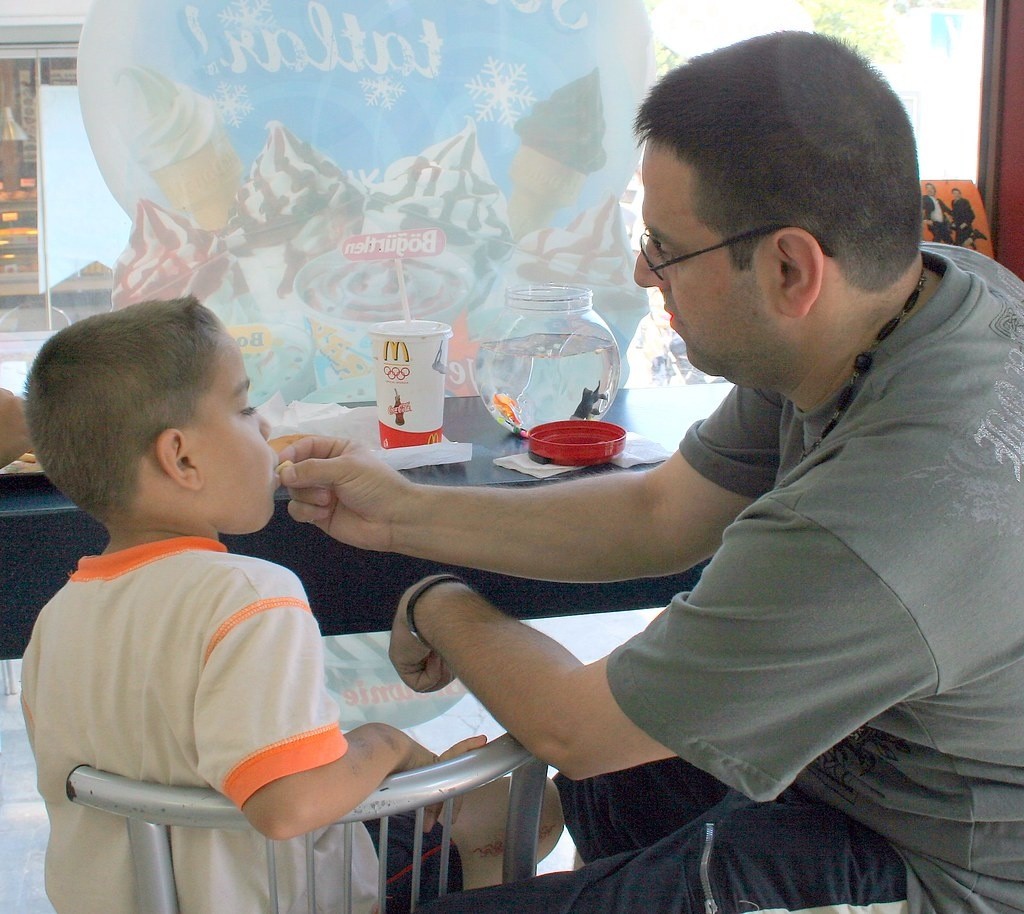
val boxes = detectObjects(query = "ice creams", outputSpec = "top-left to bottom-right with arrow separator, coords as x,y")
108,65 -> 645,400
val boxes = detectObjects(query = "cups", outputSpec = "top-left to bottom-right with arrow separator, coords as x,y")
367,320 -> 454,451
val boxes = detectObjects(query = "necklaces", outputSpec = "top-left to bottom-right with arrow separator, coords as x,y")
797,269 -> 926,463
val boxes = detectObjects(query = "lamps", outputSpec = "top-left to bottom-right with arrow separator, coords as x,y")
0,105 -> 28,142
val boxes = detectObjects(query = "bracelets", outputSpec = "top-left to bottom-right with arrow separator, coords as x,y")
406,576 -> 463,646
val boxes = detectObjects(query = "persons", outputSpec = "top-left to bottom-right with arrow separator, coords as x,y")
19,292 -> 566,914
271,30 -> 1024,914
0,387 -> 31,469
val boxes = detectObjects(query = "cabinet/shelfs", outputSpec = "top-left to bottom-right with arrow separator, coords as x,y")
1,199 -> 40,285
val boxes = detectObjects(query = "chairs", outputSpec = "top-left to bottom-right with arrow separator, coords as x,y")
65,730 -> 551,914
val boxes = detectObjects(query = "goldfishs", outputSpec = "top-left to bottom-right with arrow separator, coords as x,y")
493,393 -> 521,426
571,378 -> 608,420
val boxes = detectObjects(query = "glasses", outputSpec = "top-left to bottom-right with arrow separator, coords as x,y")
640,225 -> 832,281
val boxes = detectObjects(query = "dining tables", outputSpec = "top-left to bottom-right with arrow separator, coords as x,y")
1,393 -> 714,659
